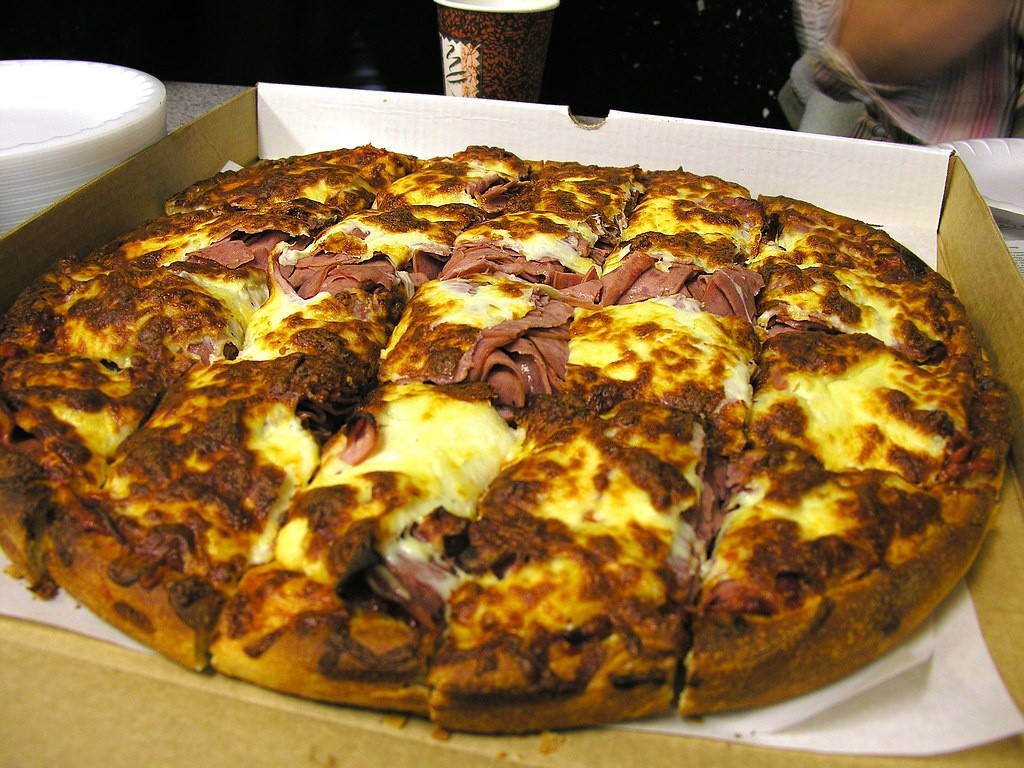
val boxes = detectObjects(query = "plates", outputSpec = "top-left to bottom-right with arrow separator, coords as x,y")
0,60 -> 167,233
919,138 -> 1024,227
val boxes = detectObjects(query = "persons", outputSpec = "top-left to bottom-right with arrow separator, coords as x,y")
774,1 -> 1024,147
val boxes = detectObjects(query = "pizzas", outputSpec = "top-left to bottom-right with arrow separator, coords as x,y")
1,143 -> 1004,729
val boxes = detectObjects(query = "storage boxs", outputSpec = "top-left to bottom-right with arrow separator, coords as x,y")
0,83 -> 1024,768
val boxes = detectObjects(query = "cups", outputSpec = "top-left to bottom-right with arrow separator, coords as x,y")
433,0 -> 560,103
